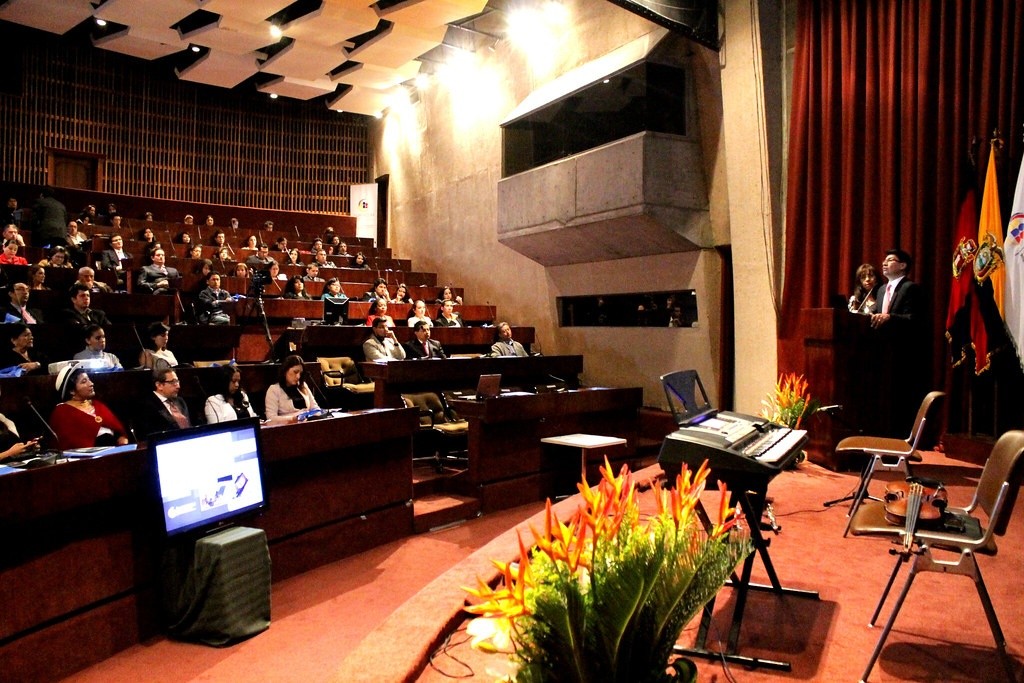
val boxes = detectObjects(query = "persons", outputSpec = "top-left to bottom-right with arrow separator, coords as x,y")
140,368 -> 191,436
0,192 -> 433,327
48,365 -> 130,453
0,390 -> 40,463
265,355 -> 321,420
403,321 -> 447,359
0,323 -> 48,375
434,299 -> 463,327
848,263 -> 883,315
362,318 -> 406,362
490,322 -> 529,357
73,324 -> 124,373
435,285 -> 461,305
868,249 -> 921,436
137,322 -> 179,370
204,364 -> 258,424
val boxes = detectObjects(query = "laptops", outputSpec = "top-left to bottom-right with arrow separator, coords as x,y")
458,374 -> 501,400
828,295 -> 849,313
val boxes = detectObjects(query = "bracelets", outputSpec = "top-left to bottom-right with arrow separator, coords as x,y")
36,361 -> 41,369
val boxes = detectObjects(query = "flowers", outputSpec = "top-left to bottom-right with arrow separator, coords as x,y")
462,451 -> 759,683
758,371 -> 821,429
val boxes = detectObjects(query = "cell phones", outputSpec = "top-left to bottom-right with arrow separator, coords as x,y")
30,437 -> 40,443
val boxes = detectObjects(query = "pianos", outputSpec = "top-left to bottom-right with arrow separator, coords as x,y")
657,407 -> 819,672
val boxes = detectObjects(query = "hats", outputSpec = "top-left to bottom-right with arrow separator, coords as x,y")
55,364 -> 84,404
149,322 -> 170,337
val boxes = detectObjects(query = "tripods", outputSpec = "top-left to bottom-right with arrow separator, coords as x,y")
824,412 -> 885,507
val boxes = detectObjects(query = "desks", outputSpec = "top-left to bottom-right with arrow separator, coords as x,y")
0,211 -> 645,566
540,431 -> 629,499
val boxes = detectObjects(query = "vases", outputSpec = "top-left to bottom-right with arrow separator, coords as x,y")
787,455 -> 799,469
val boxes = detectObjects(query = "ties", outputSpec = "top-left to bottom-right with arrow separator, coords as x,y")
20,307 -> 36,324
884,284 -> 892,314
423,341 -> 430,357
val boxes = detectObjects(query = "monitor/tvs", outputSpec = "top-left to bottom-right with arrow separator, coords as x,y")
147,416 -> 271,539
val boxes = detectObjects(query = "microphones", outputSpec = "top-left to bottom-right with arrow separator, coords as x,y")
533,335 -> 544,357
126,218 -> 431,423
857,283 -> 878,313
548,374 -> 567,390
817,404 -> 845,412
24,396 -> 68,464
486,301 -> 497,327
450,282 -> 457,297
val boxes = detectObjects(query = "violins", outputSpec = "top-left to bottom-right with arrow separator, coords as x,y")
885,477 -> 949,559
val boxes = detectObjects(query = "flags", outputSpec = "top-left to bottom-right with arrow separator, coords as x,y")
1003,151 -> 1024,374
970,142 -> 1005,375
945,148 -> 978,372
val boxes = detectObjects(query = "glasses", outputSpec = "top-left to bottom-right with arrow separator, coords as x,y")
161,379 -> 180,387
882,258 -> 901,264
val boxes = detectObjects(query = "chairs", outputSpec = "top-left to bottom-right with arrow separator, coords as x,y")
403,392 -> 471,473
860,431 -> 1024,683
836,391 -> 946,537
316,356 -> 376,410
